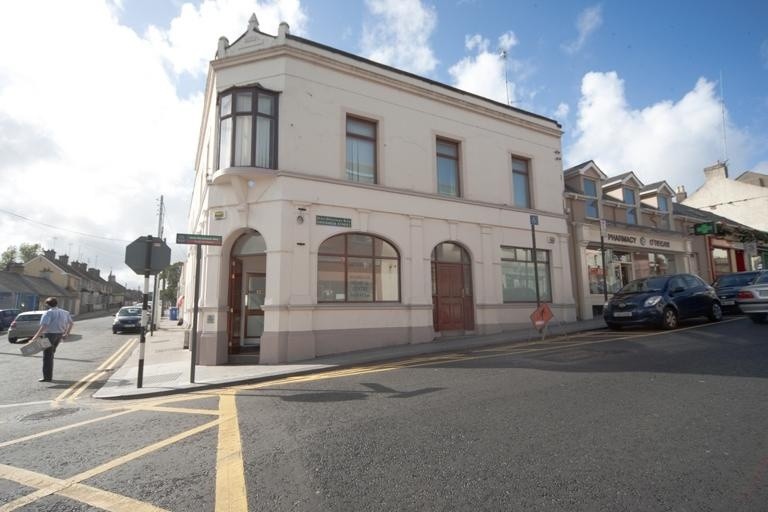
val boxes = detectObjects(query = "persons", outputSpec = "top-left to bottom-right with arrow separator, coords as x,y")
29,297 -> 74,382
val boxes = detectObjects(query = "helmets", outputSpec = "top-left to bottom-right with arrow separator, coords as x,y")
44,297 -> 57,305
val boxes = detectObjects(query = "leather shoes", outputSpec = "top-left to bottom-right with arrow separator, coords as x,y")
38,379 -> 51,382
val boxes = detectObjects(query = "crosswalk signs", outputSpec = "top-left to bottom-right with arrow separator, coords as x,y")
530,304 -> 553,331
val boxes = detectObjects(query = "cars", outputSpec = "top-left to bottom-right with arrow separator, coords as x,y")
112,300 -> 154,334
603,270 -> 768,330
1,308 -> 48,343
